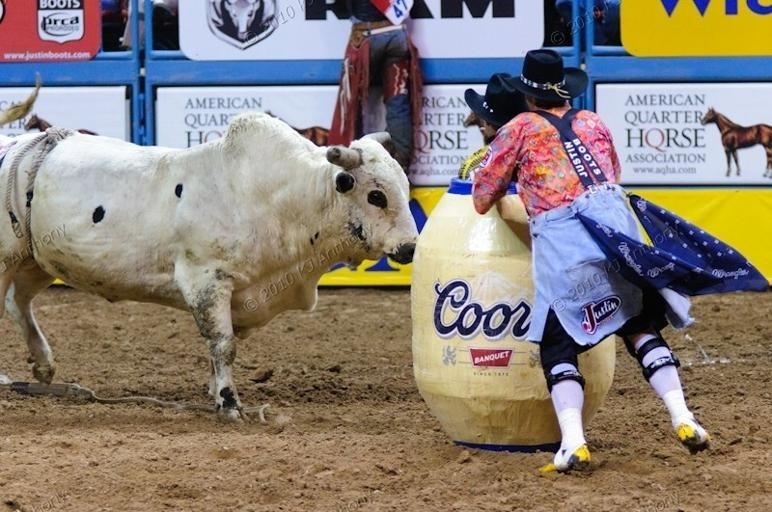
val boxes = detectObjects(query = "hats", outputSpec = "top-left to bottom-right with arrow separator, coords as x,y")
464,72 -> 530,127
503,49 -> 588,100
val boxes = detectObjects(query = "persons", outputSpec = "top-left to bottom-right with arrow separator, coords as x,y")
472,49 -> 710,471
333,0 -> 415,175
458,73 -> 529,180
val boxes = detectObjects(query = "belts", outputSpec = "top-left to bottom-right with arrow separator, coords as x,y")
363,22 -> 405,37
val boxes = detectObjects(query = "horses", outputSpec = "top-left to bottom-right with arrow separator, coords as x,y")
701,106 -> 772,178
25,114 -> 98,135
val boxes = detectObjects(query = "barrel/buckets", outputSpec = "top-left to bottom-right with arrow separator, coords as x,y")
410,177 -> 615,450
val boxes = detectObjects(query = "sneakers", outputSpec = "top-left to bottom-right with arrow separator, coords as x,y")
672,416 -> 711,453
538,444 -> 592,471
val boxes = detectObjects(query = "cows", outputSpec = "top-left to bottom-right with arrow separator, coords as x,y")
0,71 -> 419,425
